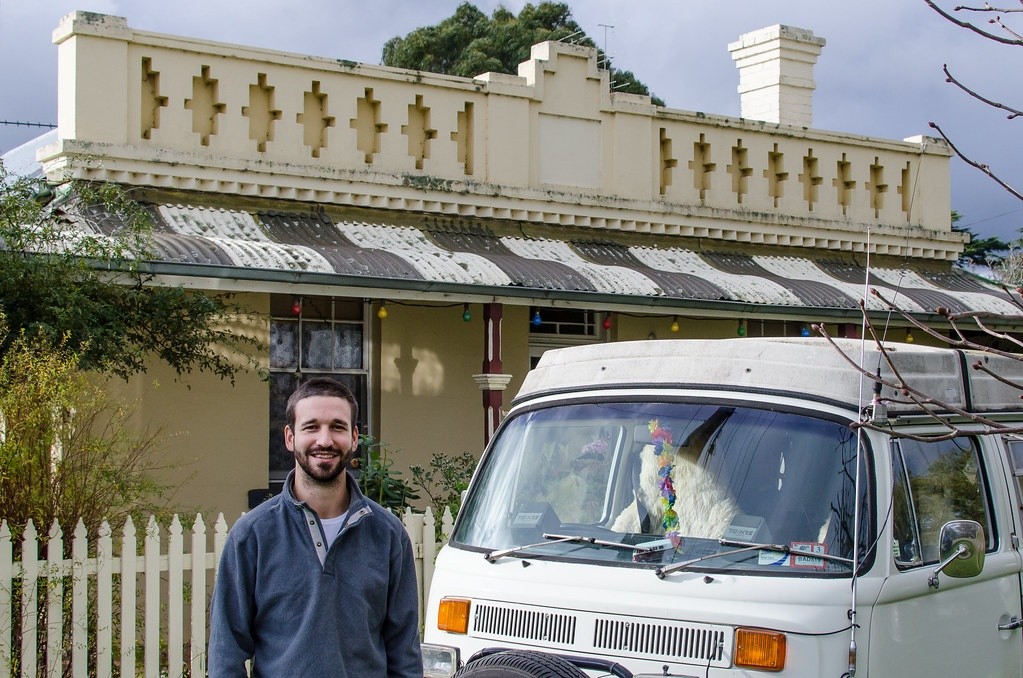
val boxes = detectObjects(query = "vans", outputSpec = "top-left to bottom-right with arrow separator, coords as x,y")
420,338 -> 1023,678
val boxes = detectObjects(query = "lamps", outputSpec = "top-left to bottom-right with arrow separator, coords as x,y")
272,282 -> 925,351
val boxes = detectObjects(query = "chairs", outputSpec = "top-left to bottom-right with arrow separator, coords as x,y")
818,431 -> 964,566
609,426 -> 750,546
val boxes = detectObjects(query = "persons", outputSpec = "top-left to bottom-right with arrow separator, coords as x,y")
207,379 -> 425,678
